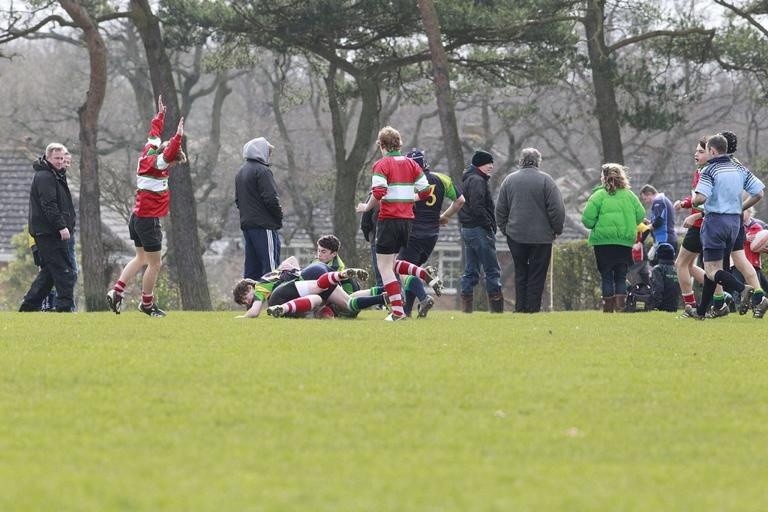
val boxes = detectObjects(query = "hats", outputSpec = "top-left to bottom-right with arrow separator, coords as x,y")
405,150 -> 424,159
472,150 -> 494,166
657,243 -> 676,260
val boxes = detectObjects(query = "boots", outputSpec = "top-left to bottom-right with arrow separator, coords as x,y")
460,294 -> 473,312
613,294 -> 626,313
602,294 -> 615,312
489,292 -> 503,312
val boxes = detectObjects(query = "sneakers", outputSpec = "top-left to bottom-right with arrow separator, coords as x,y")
342,268 -> 369,281
676,285 -> 768,321
267,306 -> 285,318
107,290 -> 122,314
383,266 -> 442,322
138,303 -> 167,317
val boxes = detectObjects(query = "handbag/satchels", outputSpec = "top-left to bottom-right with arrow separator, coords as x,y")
633,243 -> 644,260
627,282 -> 654,312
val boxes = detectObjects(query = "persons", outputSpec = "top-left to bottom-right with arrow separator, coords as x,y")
581,131 -> 768,320
232,235 -> 407,320
107,94 -> 187,317
456,151 -> 503,313
18,142 -> 78,311
355,126 -> 465,320
58,152 -> 78,310
496,148 -> 565,314
235,137 -> 284,310
